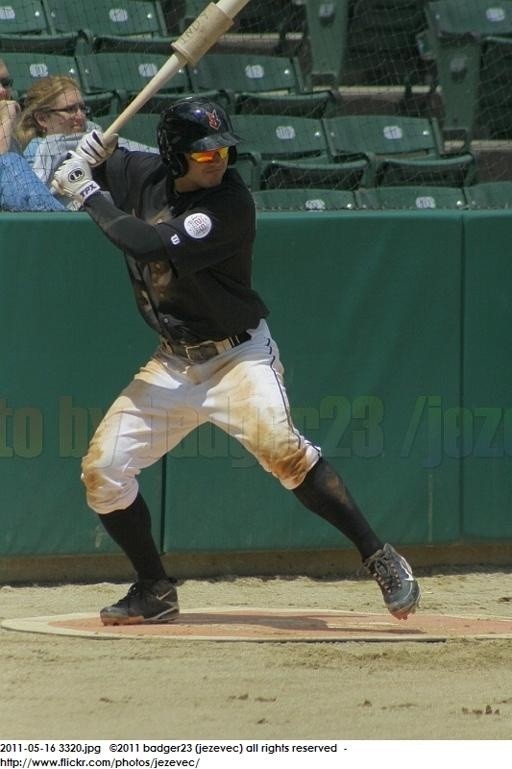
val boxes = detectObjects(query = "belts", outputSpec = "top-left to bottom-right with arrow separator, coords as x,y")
154,316 -> 265,361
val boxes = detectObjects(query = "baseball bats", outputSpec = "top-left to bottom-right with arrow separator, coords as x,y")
49,1 -> 248,197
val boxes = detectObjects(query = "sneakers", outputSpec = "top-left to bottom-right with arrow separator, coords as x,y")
359,543 -> 421,621
99,579 -> 178,623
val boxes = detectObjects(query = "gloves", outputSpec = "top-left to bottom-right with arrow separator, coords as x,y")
49,129 -> 118,205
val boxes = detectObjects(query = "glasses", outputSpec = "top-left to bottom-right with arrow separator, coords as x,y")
44,102 -> 88,114
0,78 -> 15,88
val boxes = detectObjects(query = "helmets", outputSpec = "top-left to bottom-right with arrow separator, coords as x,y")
158,93 -> 236,180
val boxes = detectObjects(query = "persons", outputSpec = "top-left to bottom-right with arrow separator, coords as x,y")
0,57 -> 25,103
18,74 -> 159,210
51,102 -> 418,627
2,101 -> 69,211
